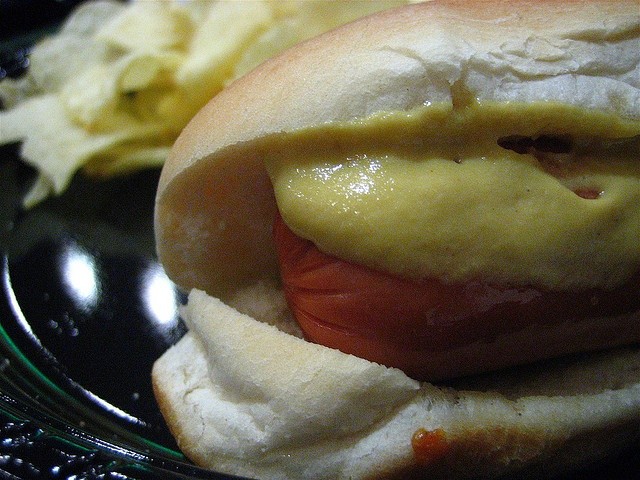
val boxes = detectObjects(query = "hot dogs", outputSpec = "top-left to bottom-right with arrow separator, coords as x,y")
150,2 -> 640,476
